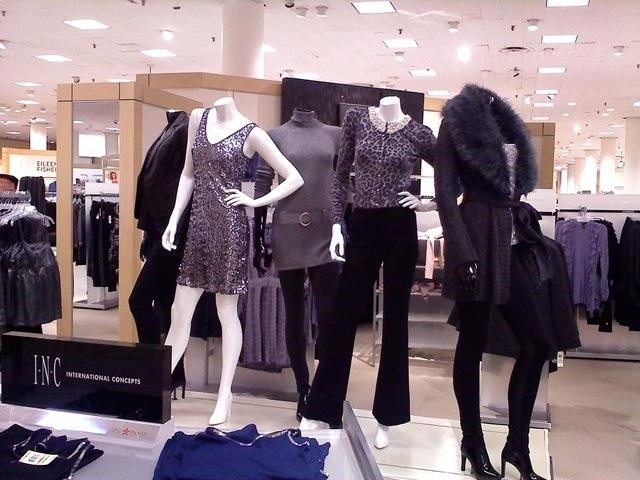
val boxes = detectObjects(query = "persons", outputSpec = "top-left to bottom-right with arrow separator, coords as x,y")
251,107 -> 349,423
434,82 -> 551,480
109,172 -> 118,184
128,106 -> 193,400
163,96 -> 305,425
296,95 -> 464,450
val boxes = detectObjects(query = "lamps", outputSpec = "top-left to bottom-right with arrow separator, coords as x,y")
613,46 -> 624,54
295,7 -> 308,18
528,19 -> 538,29
315,6 -> 328,17
448,21 -> 459,31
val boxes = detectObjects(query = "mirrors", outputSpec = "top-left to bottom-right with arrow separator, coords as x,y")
72,101 -> 120,341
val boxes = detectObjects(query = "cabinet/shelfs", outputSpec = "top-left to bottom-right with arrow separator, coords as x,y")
372,264 -> 457,367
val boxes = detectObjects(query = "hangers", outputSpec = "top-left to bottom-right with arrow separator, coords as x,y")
575,204 -> 601,223
73,192 -> 84,206
0,190 -> 55,226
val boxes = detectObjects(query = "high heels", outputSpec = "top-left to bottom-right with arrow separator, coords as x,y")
461,438 -> 546,480
171,371 -> 185,399
208,393 -> 233,425
296,385 -> 311,421
375,425 -> 389,449
329,420 -> 344,428
